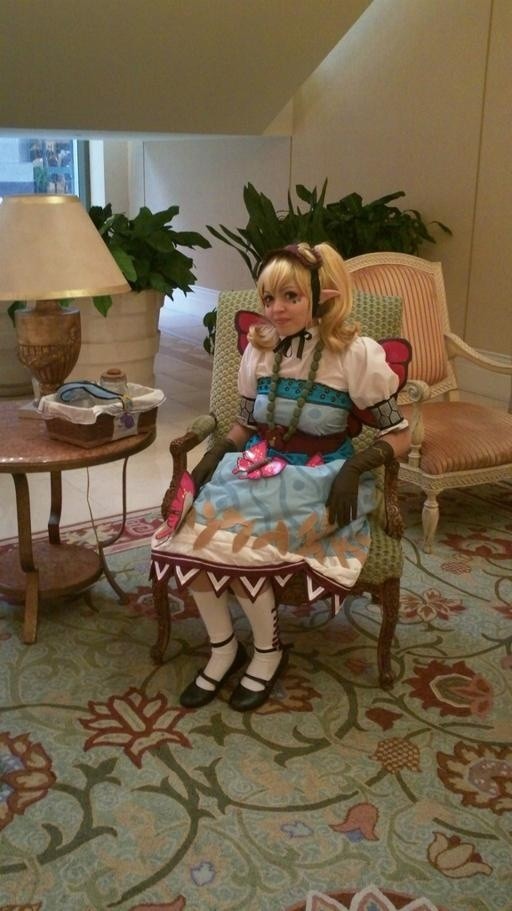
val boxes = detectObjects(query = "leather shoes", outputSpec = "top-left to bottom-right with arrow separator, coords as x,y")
228,641 -> 295,711
179,632 -> 248,711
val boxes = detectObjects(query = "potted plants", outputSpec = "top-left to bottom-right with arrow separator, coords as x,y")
5,203 -> 214,406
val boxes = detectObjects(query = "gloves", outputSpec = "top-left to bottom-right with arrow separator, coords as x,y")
190,436 -> 238,501
324,439 -> 396,527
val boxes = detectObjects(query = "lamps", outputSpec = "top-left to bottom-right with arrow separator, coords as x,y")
0,195 -> 132,418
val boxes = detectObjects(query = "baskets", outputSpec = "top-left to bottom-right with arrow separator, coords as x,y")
37,380 -> 168,449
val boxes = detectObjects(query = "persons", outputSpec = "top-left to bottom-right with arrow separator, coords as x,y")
148,240 -> 412,712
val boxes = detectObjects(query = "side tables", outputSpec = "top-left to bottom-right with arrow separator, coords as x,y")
0,393 -> 157,645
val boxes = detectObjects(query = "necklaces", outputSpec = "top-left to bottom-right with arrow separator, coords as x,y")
262,337 -> 326,451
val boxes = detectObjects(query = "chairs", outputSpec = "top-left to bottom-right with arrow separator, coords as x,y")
329,250 -> 512,556
147,288 -> 411,692
